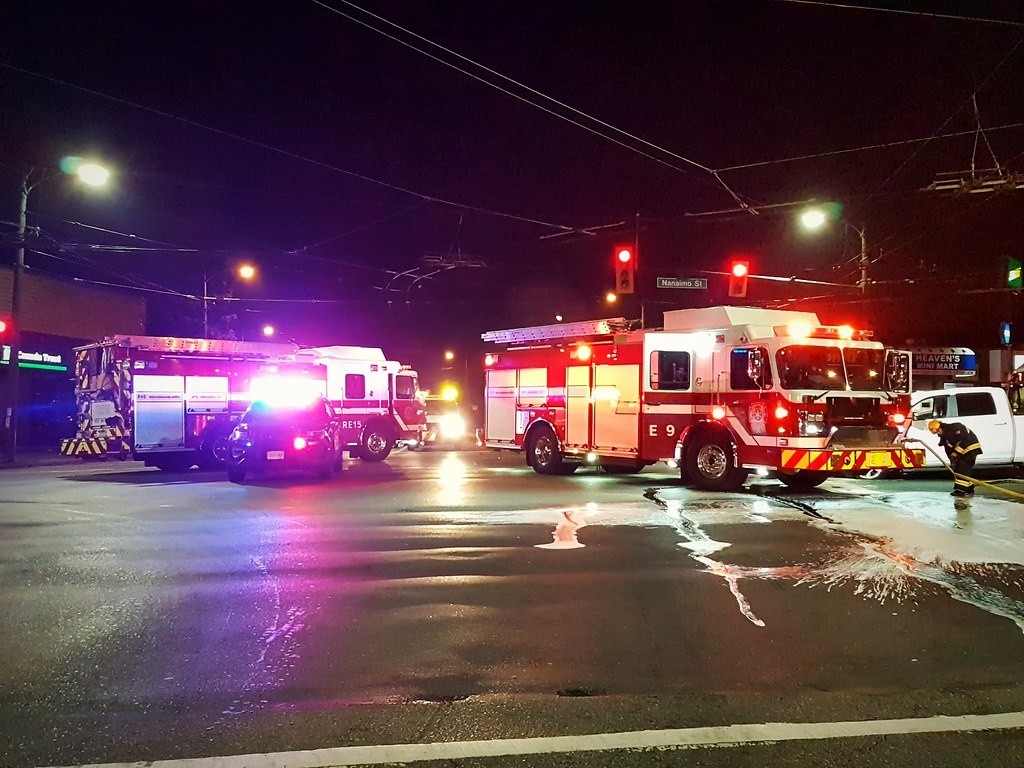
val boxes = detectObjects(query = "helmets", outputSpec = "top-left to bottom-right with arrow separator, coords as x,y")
928,420 -> 942,434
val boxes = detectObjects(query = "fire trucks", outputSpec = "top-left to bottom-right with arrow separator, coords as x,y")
57,332 -> 434,474
475,306 -> 927,492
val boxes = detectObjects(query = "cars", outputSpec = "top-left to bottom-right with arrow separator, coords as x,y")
225,392 -> 346,478
410,397 -> 466,453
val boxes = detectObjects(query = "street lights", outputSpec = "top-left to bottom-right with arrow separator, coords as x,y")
4,163 -> 106,464
801,211 -> 871,331
204,266 -> 255,338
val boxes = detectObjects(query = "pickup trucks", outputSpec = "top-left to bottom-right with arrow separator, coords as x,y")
859,386 -> 1023,480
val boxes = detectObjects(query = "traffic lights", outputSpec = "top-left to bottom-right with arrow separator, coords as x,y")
614,245 -> 635,295
729,260 -> 750,298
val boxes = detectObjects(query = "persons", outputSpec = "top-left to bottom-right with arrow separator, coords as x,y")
928,420 -> 983,496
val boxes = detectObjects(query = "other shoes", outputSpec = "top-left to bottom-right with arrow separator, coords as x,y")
950,491 -> 965,496
965,488 -> 974,493
